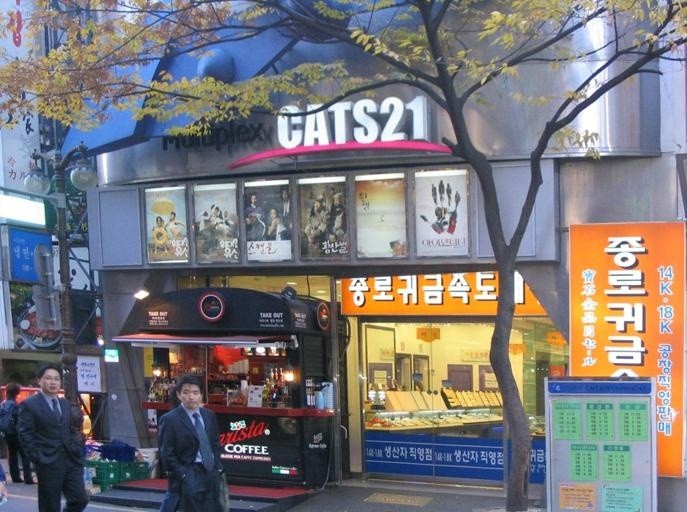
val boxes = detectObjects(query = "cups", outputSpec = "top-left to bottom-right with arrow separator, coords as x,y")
226,357 -> 249,374
313,380 -> 333,409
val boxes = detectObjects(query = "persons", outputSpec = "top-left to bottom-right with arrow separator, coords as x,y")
152,185 -> 347,255
156,376 -> 230,512
0,362 -> 89,512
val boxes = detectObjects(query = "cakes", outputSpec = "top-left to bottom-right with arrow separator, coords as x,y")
364,412 -> 502,430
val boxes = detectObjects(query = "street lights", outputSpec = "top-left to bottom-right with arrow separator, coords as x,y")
23,140 -> 101,442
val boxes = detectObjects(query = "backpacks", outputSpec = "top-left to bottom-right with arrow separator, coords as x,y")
0,403 -> 16,432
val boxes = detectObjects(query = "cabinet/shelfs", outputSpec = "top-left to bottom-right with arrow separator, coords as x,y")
361,404 -> 505,434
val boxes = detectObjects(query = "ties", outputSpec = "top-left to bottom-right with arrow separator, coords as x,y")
192,413 -> 215,472
52,399 -> 61,424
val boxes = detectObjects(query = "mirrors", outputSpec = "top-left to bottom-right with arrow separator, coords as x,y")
360,316 -> 524,412
365,324 -> 399,400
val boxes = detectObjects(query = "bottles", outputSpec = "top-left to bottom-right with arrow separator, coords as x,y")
268,365 -> 283,383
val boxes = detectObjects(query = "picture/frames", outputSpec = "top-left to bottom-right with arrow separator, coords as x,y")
355,169 -> 411,260
411,167 -> 472,259
296,173 -> 351,262
139,180 -> 192,265
192,180 -> 242,265
243,174 -> 297,264
